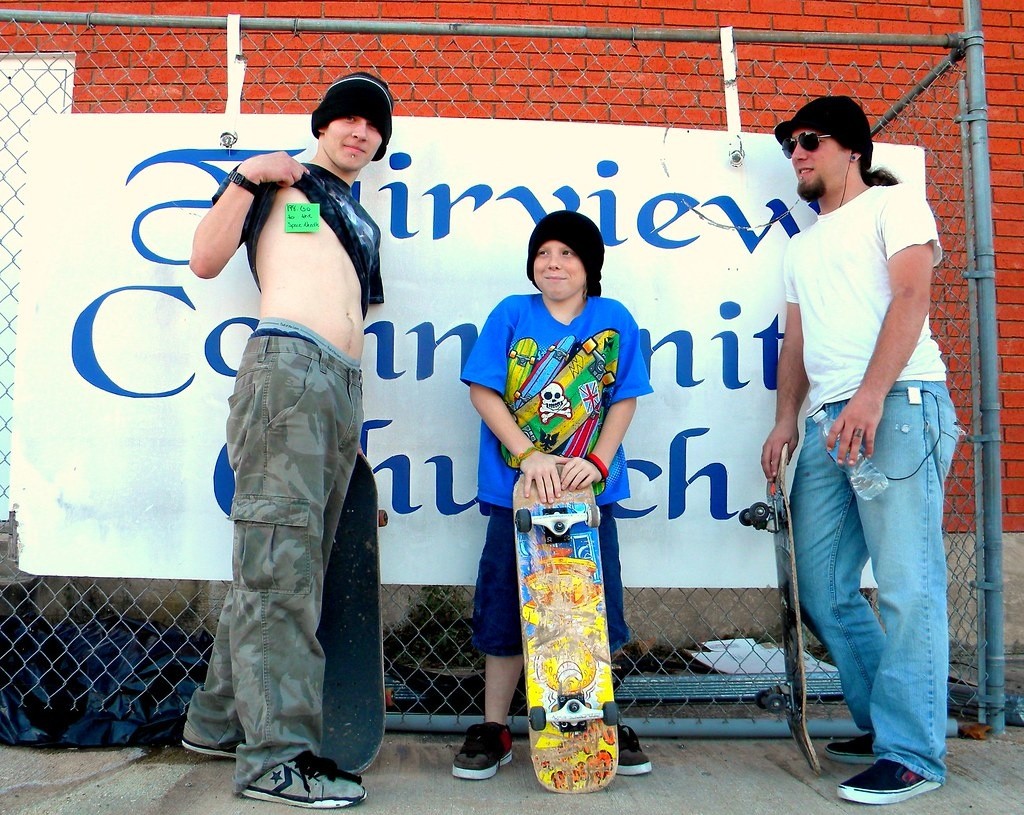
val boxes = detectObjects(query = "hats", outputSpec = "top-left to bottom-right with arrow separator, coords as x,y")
526,210 -> 603,296
775,96 -> 873,170
311,72 -> 393,161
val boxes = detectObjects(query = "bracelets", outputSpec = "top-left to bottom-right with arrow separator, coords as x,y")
515,446 -> 541,465
229,170 -> 259,196
584,453 -> 608,482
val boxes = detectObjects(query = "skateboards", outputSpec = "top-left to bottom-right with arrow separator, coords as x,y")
511,463 -> 621,793
316,454 -> 396,776
739,444 -> 822,777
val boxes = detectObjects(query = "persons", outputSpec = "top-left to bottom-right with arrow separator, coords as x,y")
453,208 -> 653,778
182,72 -> 395,807
760,95 -> 957,803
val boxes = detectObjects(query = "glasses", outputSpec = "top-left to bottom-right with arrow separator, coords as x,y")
782,132 -> 833,160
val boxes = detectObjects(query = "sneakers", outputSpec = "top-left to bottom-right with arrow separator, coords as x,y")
837,746 -> 941,804
824,733 -> 875,763
182,735 -> 240,758
240,746 -> 367,810
613,722 -> 652,776
453,719 -> 514,779
